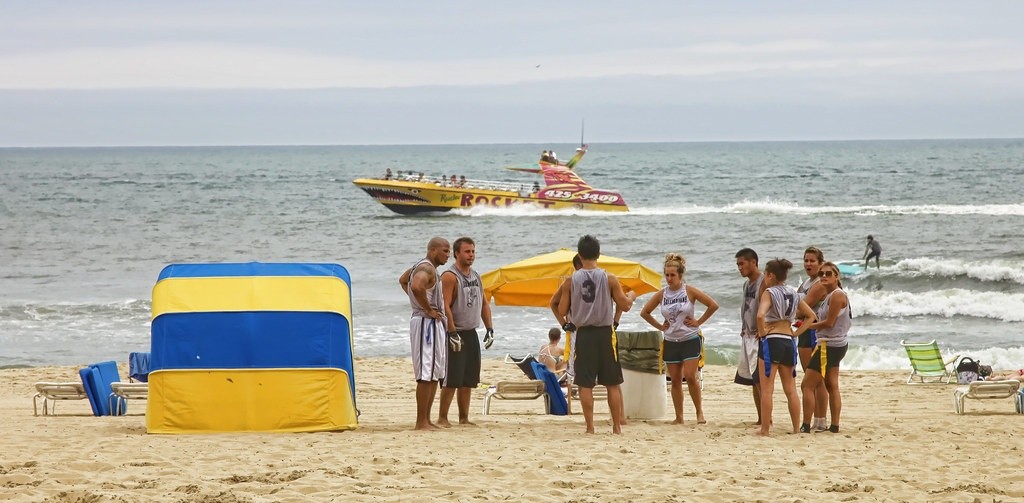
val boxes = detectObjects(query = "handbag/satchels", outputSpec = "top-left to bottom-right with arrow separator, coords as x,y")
957,357 -> 979,384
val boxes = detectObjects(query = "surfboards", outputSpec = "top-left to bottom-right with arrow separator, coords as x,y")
837,264 -> 873,275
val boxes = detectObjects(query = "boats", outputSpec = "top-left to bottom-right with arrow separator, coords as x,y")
353,118 -> 630,216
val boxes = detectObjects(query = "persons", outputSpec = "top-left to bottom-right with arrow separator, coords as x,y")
440,238 -> 495,428
735,248 -> 772,426
550,234 -> 636,435
384,168 -> 466,189
540,150 -> 557,162
800,262 -> 851,434
797,246 -> 830,434
540,328 -> 569,382
755,259 -> 817,438
397,237 -> 450,431
640,254 -> 719,426
862,234 -> 882,272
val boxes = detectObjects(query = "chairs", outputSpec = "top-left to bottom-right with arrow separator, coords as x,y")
567,382 -> 611,415
504,352 -> 574,386
954,380 -> 1024,415
899,340 -> 960,384
108,381 -> 149,416
33,382 -> 90,417
482,380 -> 551,415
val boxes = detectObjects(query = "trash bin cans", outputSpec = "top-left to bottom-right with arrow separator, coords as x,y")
615,327 -> 668,419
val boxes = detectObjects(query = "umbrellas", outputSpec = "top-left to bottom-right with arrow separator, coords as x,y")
478,248 -> 662,416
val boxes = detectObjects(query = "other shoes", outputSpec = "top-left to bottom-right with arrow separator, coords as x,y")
812,423 -> 828,432
800,424 -> 810,433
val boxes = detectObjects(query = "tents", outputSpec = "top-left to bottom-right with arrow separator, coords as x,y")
146,261 -> 362,435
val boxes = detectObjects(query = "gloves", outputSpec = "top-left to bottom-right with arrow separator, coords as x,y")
483,328 -> 495,349
613,321 -> 619,330
448,331 -> 464,352
562,322 -> 577,333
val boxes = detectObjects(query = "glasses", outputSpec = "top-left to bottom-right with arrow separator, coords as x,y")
818,270 -> 836,277
